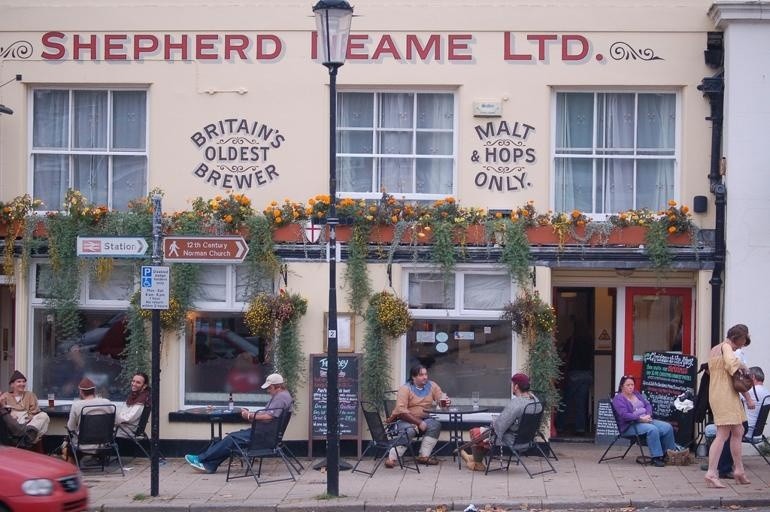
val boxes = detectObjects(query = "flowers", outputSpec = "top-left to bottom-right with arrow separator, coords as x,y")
265,198 -> 303,226
335,197 -> 354,218
571,210 -> 586,227
619,208 -> 653,226
359,188 -> 430,239
210,191 -> 252,231
658,199 -> 689,233
61,188 -> 106,223
510,200 -> 534,226
434,197 -> 457,222
304,195 -> 330,222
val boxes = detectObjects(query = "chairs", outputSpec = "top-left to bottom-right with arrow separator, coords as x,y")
143,438 -> 144,439
249,401 -> 304,475
64,404 -> 126,477
598,393 -> 653,467
225,408 -> 296,488
484,401 -> 559,479
351,400 -> 421,479
740,394 -> 770,466
114,403 -> 166,463
11,433 -> 39,449
381,389 -> 420,466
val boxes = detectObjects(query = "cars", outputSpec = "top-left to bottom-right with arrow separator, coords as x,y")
195,328 -> 259,364
0,445 -> 89,512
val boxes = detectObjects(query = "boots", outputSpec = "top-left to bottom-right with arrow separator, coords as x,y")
701,436 -> 715,469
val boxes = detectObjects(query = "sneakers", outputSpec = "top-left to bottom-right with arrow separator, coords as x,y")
185,454 -> 199,464
467,461 -> 485,471
190,461 -> 215,473
385,460 -> 394,468
651,457 -> 665,466
418,458 -> 439,465
461,450 -> 473,463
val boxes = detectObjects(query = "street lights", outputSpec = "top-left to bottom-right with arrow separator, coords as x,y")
311,0 -> 357,496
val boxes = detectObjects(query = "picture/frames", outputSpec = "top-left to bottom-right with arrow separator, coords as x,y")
323,311 -> 355,353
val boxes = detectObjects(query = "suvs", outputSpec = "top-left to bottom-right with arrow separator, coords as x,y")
56,313 -> 127,354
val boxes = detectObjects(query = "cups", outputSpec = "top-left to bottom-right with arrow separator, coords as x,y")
48,394 -> 54,408
472,392 -> 479,409
439,393 -> 446,408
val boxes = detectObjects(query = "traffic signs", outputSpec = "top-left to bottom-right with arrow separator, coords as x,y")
139,265 -> 170,309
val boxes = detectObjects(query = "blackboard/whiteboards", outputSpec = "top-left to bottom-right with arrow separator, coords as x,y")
594,400 -> 630,446
307,353 -> 362,461
639,351 -> 698,420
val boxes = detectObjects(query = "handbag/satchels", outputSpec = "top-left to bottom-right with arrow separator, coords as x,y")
721,342 -> 753,392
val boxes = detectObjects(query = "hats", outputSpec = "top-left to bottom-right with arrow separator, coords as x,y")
78,377 -> 96,390
511,373 -> 529,386
260,374 -> 284,390
9,370 -> 26,384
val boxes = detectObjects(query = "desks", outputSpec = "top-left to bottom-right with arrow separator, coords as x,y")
40,404 -> 73,416
169,407 -> 246,442
681,407 -> 710,459
423,406 -> 489,470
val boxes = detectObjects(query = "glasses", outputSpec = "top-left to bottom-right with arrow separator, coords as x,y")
623,375 -> 632,378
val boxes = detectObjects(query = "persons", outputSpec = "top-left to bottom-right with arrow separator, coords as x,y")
384,363 -> 451,467
67,377 -> 115,454
185,373 -> 293,473
703,323 -> 756,488
116,373 -> 149,438
461,373 -> 542,471
612,375 -> 676,467
0,370 -> 50,450
718,366 -> 765,478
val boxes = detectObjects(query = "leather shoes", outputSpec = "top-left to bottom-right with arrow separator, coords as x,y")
719,472 -> 734,479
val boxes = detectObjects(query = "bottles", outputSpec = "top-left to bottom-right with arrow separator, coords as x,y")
228,392 -> 234,411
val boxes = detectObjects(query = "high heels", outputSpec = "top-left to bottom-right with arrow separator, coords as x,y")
704,476 -> 726,488
734,472 -> 751,485
